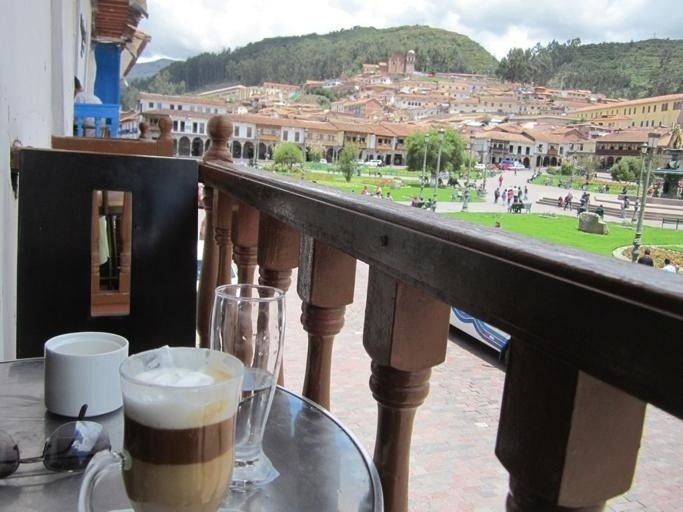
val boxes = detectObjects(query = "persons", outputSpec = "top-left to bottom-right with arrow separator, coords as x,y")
361,184 -> 393,203
356,163 -> 360,177
660,258 -> 680,273
494,166 -> 610,220
411,168 -> 487,212
618,184 -> 629,219
638,247 -> 653,267
378,172 -> 382,178
647,173 -> 664,198
74,76 -> 107,139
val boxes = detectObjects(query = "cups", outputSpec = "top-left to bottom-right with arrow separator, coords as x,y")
41,330 -> 130,418
77,346 -> 245,512
210,283 -> 287,464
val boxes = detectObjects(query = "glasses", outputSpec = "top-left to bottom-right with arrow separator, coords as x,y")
0,404 -> 112,481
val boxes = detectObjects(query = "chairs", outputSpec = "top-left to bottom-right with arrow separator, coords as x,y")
17,146 -> 199,358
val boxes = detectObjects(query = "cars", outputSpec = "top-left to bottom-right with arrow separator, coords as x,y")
473,159 -> 525,170
365,160 -> 382,167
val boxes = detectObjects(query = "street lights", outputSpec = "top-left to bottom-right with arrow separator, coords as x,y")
630,141 -> 649,223
418,133 -> 431,202
431,128 -> 445,213
631,129 -> 661,264
462,132 -> 476,212
